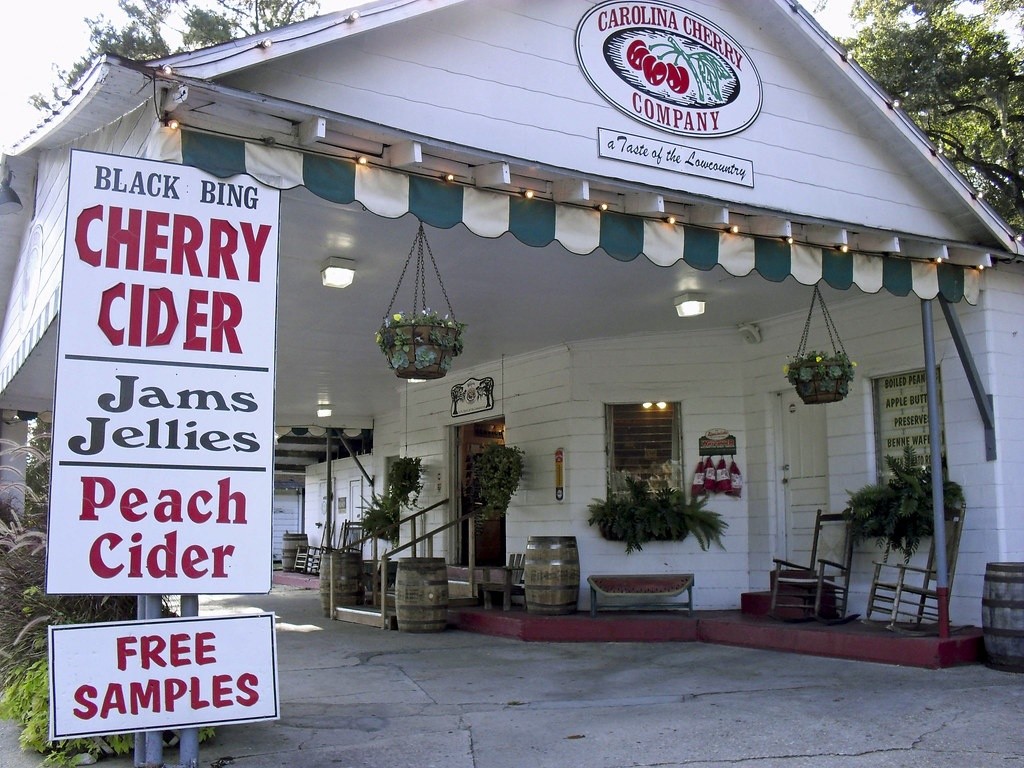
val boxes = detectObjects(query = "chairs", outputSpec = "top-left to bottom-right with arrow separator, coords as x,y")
480,555 -> 532,612
290,519 -> 364,576
765,509 -> 860,625
857,501 -> 971,628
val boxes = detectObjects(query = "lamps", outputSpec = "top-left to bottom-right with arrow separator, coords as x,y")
673,291 -> 708,317
319,256 -> 357,289
317,409 -> 333,417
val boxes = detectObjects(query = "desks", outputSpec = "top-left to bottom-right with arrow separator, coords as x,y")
588,573 -> 695,618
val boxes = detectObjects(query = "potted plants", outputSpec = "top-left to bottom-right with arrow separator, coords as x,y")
356,444 -> 528,546
588,476 -> 729,554
845,445 -> 964,564
784,348 -> 858,404
375,311 -> 465,379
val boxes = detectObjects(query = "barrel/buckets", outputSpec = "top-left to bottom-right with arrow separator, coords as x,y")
319,553 -> 365,619
524,536 -> 581,615
981,561 -> 1024,674
395,558 -> 449,633
282,534 -> 309,573
